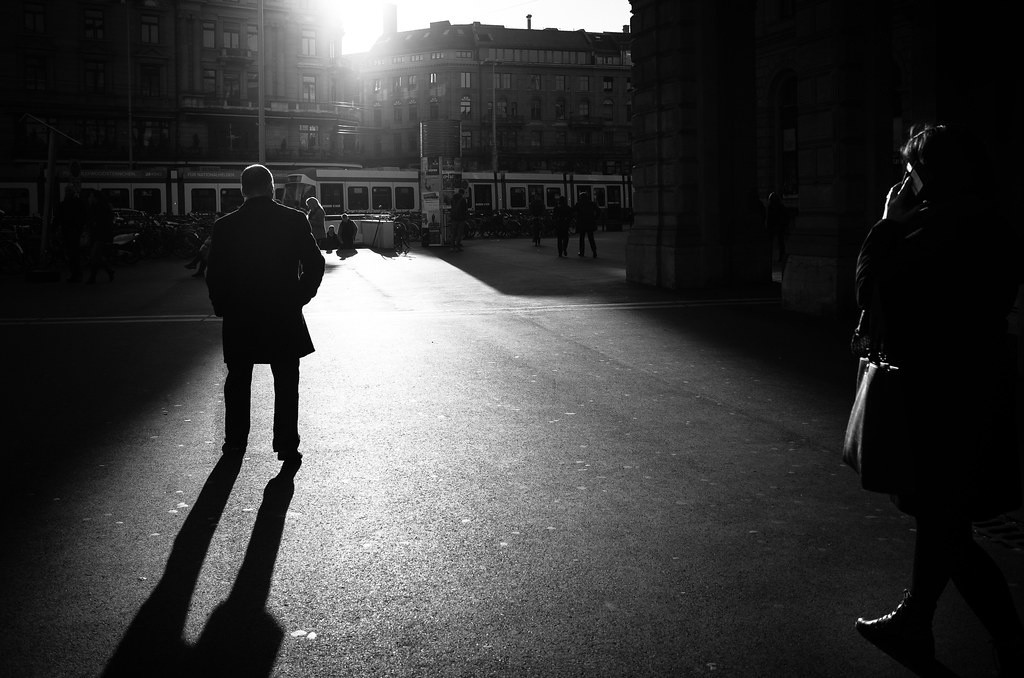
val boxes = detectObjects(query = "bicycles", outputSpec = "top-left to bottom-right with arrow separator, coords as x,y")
375,208 -> 578,248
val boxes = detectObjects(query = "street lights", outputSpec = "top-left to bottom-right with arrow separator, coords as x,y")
482,58 -> 506,172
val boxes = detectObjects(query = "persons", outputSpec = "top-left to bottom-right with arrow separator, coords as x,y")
326,226 -> 338,240
337,213 -> 358,248
573,192 -> 600,258
51,186 -> 87,284
205,163 -> 325,458
527,192 -> 543,242
840,122 -> 1024,678
306,197 -> 327,246
552,196 -> 574,257
182,212 -> 225,278
449,188 -> 468,252
87,191 -> 115,285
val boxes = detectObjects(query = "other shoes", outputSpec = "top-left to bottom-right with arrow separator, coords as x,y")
184,263 -> 196,269
108,270 -> 115,282
988,624 -> 1024,677
564,251 -> 567,255
592,253 -> 597,257
854,589 -> 936,670
192,271 -> 204,277
224,437 -> 246,448
456,244 -> 464,247
578,252 -> 583,256
86,278 -> 96,284
559,252 -> 561,257
278,451 -> 301,460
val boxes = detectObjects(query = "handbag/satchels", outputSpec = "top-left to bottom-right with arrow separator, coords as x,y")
841,307 -> 918,497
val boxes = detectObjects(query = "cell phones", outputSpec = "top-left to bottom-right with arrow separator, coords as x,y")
908,161 -> 930,209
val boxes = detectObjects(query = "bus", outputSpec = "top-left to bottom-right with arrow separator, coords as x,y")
0,159 -> 634,236
